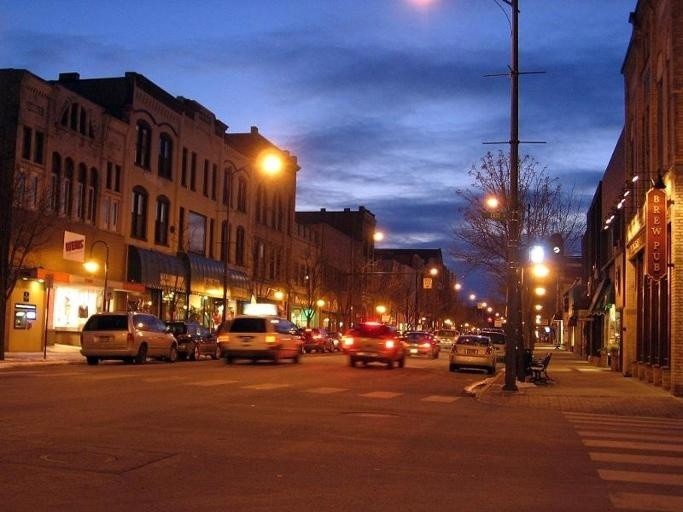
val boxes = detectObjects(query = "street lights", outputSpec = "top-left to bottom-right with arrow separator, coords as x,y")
521,243 -> 550,348
82,240 -> 109,312
413,268 -> 506,333
484,195 -> 519,390
222,153 -> 281,323
347,232 -> 384,330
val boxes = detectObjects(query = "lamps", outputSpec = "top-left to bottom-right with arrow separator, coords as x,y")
602,175 -> 656,230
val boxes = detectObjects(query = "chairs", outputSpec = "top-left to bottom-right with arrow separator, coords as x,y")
526,352 -> 552,384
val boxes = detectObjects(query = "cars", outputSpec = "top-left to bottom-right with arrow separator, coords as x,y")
401,329 -> 460,358
216,316 -> 343,364
342,324 -> 404,367
448,326 -> 506,374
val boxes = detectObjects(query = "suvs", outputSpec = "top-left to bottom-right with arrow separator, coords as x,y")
79,312 -> 222,365
